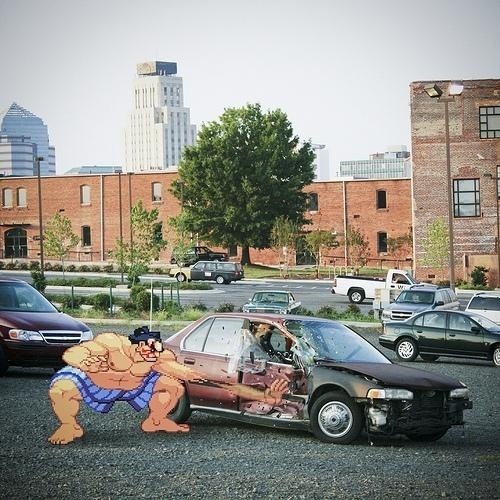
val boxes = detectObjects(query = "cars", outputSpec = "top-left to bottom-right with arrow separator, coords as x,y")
243,290 -> 304,316
147,312 -> 475,447
378,283 -> 500,365
0,277 -> 95,378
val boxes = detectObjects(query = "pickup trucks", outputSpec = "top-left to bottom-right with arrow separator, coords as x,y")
331,268 -> 422,304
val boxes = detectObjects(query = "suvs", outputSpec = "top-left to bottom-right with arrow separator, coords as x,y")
170,245 -> 244,286
